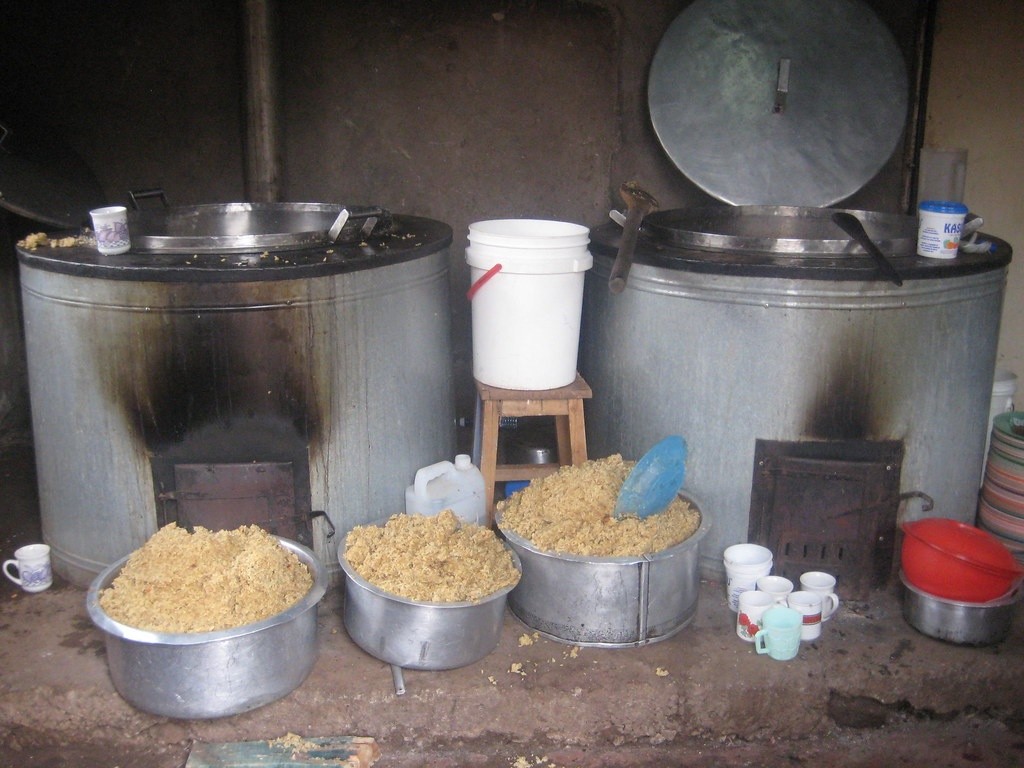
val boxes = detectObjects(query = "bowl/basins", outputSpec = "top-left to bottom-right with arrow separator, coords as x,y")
615,435 -> 688,516
901,518 -> 1024,603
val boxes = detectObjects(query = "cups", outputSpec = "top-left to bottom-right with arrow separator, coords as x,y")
736,571 -> 839,661
89,205 -> 131,256
2,543 -> 54,593
917,200 -> 968,259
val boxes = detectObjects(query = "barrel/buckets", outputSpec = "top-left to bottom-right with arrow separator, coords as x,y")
465,219 -> 594,391
722,542 -> 774,613
980,367 -> 1018,488
405,453 -> 487,528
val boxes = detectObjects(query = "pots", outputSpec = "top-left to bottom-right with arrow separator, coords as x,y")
897,568 -> 1024,647
336,514 -> 523,671
128,187 -> 383,249
85,533 -> 329,720
609,204 -> 985,259
495,489 -> 713,649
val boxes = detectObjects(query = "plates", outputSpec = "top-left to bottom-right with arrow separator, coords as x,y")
976,408 -> 1024,568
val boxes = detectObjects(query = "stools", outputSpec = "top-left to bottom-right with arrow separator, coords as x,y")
460,351 -> 593,526
